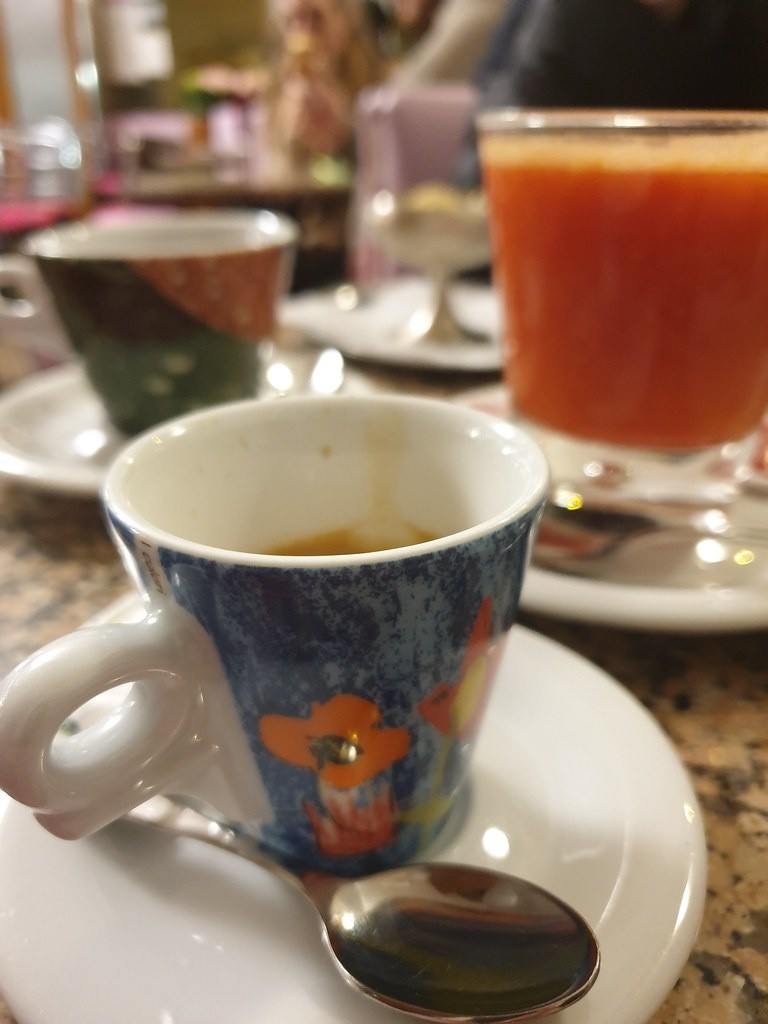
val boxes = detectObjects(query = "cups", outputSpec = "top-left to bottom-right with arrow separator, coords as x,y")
473,107 -> 768,592
14,207 -> 304,439
0,394 -> 552,878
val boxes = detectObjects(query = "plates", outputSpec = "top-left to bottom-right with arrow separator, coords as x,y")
448,381 -> 768,633
274,283 -> 503,370
0,359 -> 375,502
0,589 -> 708,1024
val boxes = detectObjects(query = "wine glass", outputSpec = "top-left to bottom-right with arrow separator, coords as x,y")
356,85 -> 494,349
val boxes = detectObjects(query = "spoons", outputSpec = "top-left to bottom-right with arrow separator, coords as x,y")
528,503 -> 768,579
123,793 -> 601,1024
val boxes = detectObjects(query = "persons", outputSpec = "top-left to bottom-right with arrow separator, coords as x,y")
263,0 -> 768,300
